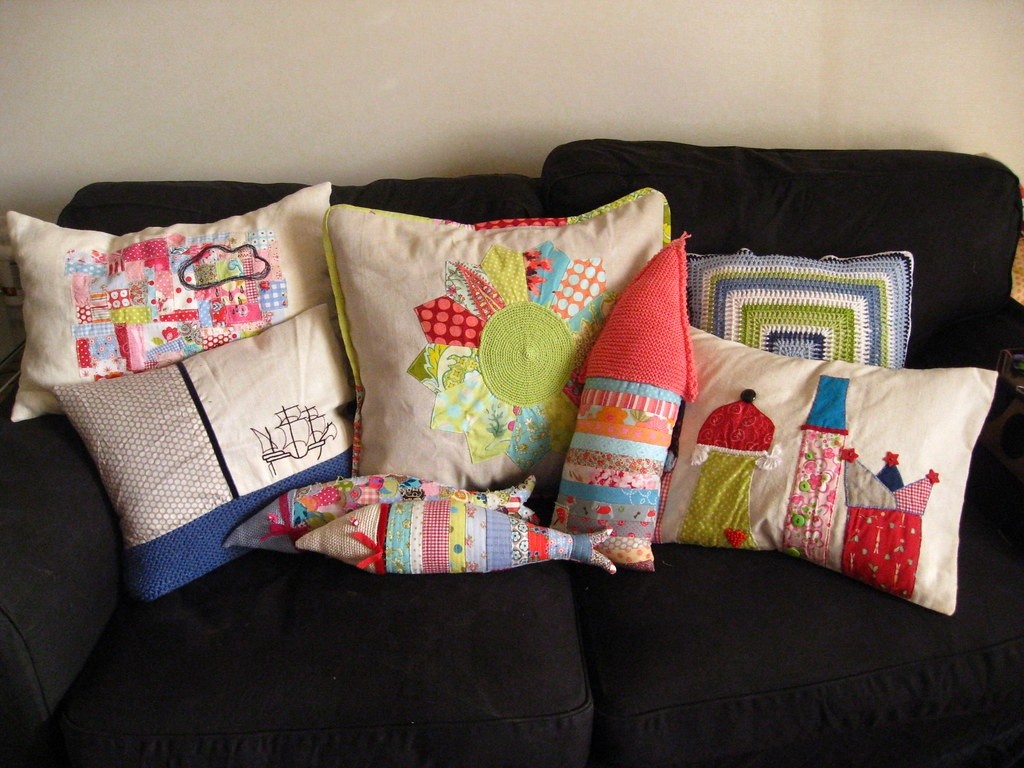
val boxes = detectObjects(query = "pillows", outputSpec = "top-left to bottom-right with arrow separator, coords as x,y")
549,234 -> 698,577
321,187 -> 672,488
223,474 -> 539,552
295,499 -> 618,575
51,303 -> 353,604
6,180 -> 333,424
685,243 -> 915,370
653,328 -> 1000,618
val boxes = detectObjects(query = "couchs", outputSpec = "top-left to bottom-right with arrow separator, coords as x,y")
0,137 -> 1024,767
